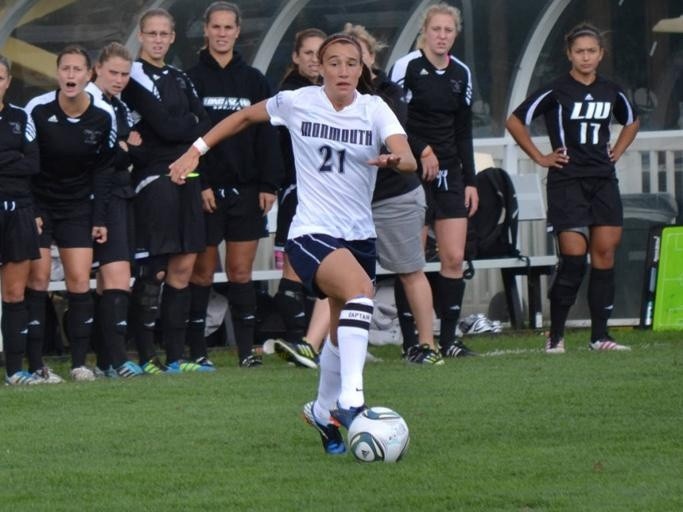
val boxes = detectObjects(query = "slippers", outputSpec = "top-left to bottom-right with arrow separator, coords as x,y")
466,168 -> 518,259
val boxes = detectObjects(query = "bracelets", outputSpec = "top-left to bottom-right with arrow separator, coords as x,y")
190,135 -> 211,157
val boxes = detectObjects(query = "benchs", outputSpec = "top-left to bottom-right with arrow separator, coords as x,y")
49,252 -> 563,353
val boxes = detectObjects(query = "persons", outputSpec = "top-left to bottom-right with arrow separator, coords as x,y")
92,39 -> 147,381
162,22 -> 422,455
273,25 -> 446,370
259,28 -> 333,342
119,6 -> 216,376
184,1 -> 287,369
501,21 -> 641,357
1,52 -> 46,392
388,3 -> 482,360
24,48 -> 120,385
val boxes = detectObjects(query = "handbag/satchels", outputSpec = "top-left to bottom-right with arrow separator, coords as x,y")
190,135 -> 211,157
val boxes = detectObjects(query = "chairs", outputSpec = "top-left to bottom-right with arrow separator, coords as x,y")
69,366 -> 96,382
140,357 -> 181,375
545,337 -> 565,354
365,352 -> 384,365
330,401 -> 370,430
4,372 -> 46,387
403,344 -> 445,366
302,401 -> 345,455
589,341 -> 630,353
164,359 -> 215,375
273,339 -> 321,370
30,368 -> 65,384
193,355 -> 215,368
239,356 -> 264,369
108,362 -> 143,378
440,342 -> 482,358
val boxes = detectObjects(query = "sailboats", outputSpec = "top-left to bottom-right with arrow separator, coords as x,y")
348,406 -> 410,463
